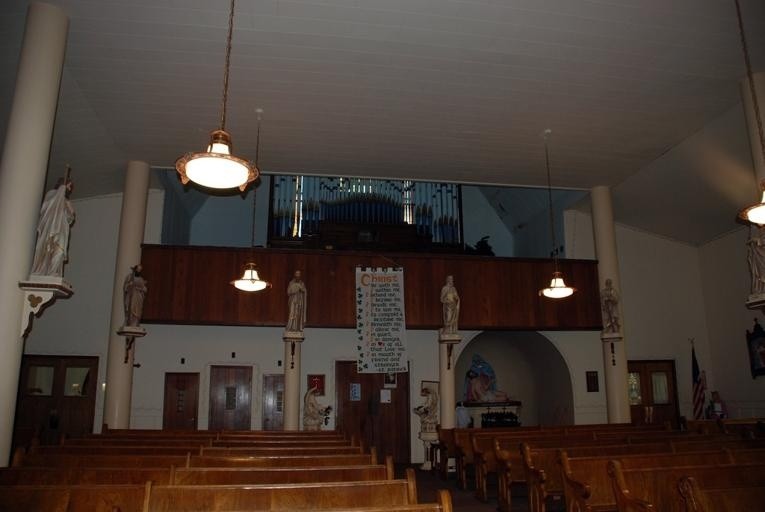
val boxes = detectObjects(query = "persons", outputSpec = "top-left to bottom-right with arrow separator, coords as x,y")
124,265 -> 148,327
32,177 -> 75,278
440,274 -> 460,334
287,270 -> 307,332
304,385 -> 323,419
746,225 -> 765,294
467,370 -> 508,402
421,388 -> 438,423
601,279 -> 621,333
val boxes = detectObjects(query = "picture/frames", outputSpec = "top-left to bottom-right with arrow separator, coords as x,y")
745,318 -> 765,379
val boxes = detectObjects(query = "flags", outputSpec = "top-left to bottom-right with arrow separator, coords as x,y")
692,347 -> 704,419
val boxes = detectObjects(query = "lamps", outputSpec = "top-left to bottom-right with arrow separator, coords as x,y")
735,1 -> 764,227
171,1 -> 275,297
535,142 -> 577,300
